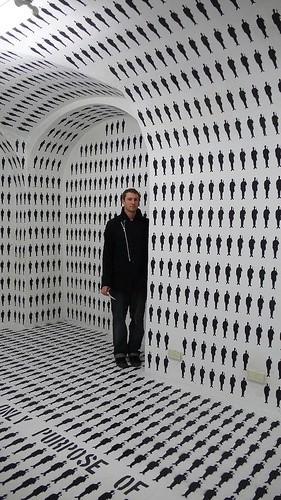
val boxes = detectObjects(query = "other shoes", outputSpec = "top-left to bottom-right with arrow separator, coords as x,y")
115,356 -> 128,368
129,356 -> 142,367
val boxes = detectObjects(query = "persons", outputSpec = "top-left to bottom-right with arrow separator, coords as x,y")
99,188 -> 149,369
